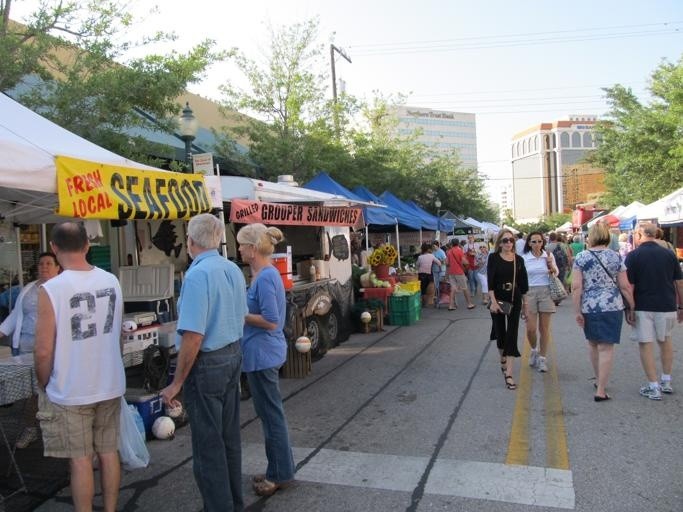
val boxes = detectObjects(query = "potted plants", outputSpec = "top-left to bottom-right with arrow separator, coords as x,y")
396,243 -> 420,284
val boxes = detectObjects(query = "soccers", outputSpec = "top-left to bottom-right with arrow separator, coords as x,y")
164,400 -> 182,417
361,311 -> 371,323
151,416 -> 175,440
295,336 -> 311,353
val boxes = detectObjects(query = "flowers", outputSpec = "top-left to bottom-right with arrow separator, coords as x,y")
367,243 -> 397,267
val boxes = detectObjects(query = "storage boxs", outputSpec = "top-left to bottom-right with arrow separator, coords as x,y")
124,295 -> 172,316
125,386 -> 163,435
270,253 -> 293,289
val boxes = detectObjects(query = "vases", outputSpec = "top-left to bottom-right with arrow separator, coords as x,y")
375,263 -> 388,280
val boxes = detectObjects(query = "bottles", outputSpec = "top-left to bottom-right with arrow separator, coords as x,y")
308,264 -> 315,274
315,267 -> 320,281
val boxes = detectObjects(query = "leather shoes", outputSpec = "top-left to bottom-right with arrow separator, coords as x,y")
254,474 -> 289,496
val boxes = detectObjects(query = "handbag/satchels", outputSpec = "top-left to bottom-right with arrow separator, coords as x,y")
548,270 -> 567,300
490,300 -> 514,314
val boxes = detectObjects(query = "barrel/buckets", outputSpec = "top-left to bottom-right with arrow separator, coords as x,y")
272,253 -> 288,287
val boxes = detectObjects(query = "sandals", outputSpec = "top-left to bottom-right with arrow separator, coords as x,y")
501,360 -> 506,372
505,375 -> 516,389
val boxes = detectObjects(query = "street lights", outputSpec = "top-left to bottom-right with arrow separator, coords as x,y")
434,196 -> 442,216
176,99 -> 198,172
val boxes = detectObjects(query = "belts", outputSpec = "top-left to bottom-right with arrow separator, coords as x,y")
496,282 -> 518,291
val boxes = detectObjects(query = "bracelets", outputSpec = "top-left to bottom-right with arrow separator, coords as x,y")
678,307 -> 683,310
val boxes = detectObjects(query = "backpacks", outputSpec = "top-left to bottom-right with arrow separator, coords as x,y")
553,243 -> 567,268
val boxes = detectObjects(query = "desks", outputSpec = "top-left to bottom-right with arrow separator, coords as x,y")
0,338 -> 158,495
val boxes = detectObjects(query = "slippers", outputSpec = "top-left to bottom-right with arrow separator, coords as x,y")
594,393 -> 610,401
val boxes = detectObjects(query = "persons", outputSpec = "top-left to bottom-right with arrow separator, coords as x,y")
162,213 -> 249,512
417,228 -> 677,311
625,222 -> 683,400
487,230 -> 528,389
571,223 -> 635,402
236,223 -> 295,496
0,253 -> 60,449
521,232 -> 560,372
34,220 -> 126,512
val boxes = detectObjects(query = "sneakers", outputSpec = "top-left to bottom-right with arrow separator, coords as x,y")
16,427 -> 39,448
659,379 -> 673,393
529,351 -> 538,366
538,357 -> 548,372
639,385 -> 662,401
447,299 -> 489,310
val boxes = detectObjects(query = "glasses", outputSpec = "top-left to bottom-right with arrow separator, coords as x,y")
531,239 -> 543,244
499,238 -> 514,244
